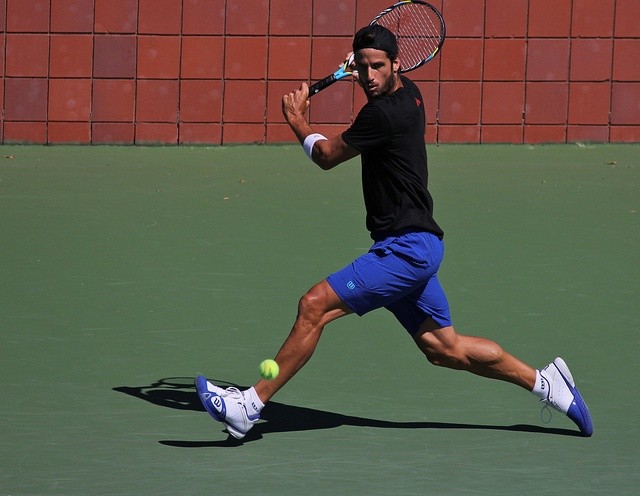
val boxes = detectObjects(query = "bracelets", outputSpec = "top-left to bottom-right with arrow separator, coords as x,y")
302,133 -> 328,161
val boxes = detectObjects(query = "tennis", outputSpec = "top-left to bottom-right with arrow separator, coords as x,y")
259,359 -> 279,380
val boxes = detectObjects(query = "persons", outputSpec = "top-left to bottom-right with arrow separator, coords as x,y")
195,24 -> 594,441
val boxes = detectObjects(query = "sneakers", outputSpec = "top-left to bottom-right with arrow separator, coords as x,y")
540,356 -> 594,438
193,376 -> 262,440
